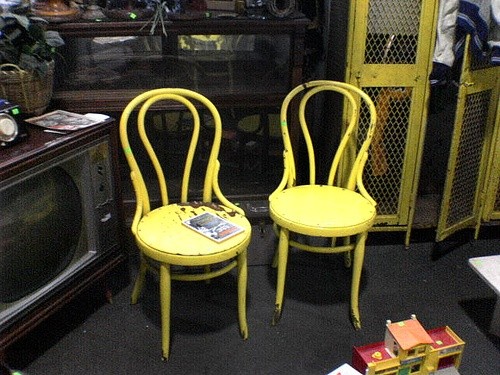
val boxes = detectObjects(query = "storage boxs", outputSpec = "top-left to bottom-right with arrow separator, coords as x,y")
205,0 -> 235,17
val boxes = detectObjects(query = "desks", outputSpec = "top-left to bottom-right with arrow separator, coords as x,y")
468,255 -> 500,336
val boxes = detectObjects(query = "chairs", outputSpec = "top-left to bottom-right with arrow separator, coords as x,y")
268,80 -> 378,330
120,88 -> 253,360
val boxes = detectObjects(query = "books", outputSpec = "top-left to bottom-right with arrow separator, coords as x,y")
181,211 -> 245,243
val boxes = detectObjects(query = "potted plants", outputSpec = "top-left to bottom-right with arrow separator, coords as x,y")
0,13 -> 66,116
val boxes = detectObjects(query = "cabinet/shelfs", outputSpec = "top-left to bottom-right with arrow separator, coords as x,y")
0,18 -> 320,351
